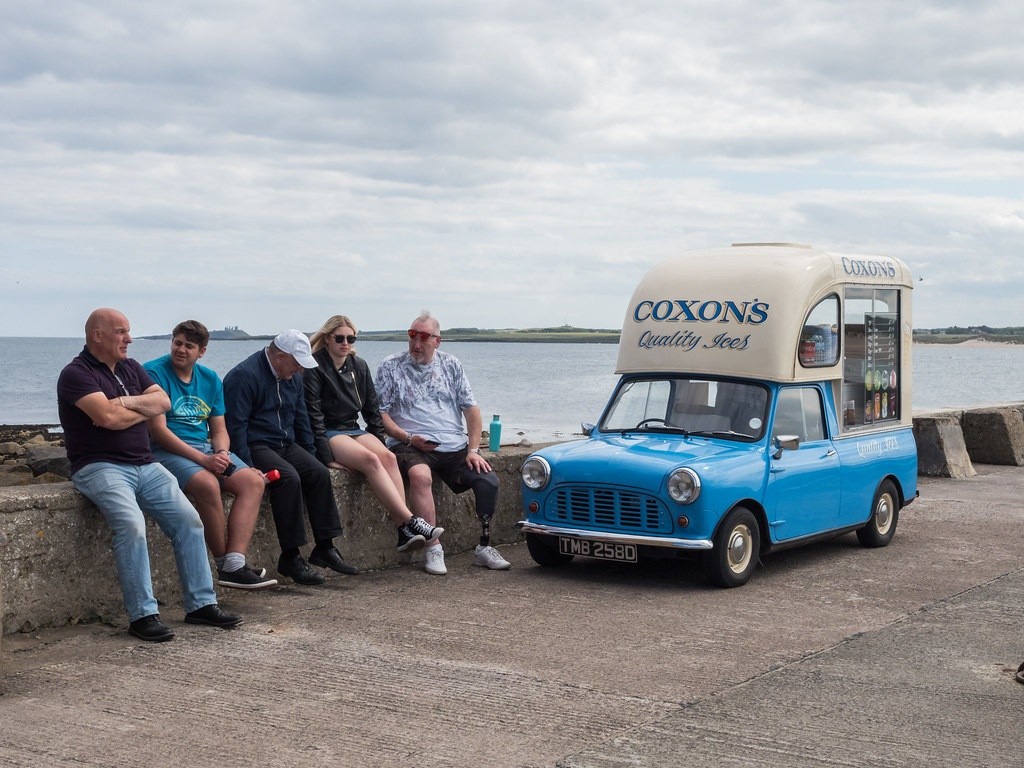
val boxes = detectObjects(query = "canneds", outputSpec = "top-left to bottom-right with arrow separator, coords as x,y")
799,341 -> 815,365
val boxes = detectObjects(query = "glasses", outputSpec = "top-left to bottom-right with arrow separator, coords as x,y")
408,329 -> 438,340
329,332 -> 357,344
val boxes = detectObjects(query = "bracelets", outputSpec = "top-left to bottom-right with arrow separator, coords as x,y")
217,449 -> 230,456
119,396 -> 125,407
406,432 -> 414,447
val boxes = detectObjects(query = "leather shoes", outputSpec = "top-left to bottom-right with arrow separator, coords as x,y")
278,553 -> 325,585
184,605 -> 243,627
128,615 -> 176,640
308,546 -> 360,574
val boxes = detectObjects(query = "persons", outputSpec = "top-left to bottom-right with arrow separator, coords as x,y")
57,308 -> 243,643
375,315 -> 510,576
301,315 -> 444,553
139,319 -> 278,590
223,328 -> 360,586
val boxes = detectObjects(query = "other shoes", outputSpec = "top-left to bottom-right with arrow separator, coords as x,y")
216,567 -> 266,578
217,569 -> 279,591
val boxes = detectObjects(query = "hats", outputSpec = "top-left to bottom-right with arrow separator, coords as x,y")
274,330 -> 320,369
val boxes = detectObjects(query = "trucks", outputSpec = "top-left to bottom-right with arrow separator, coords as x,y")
515,240 -> 919,589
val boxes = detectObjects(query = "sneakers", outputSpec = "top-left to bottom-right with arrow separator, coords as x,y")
424,547 -> 449,575
407,515 -> 445,544
397,526 -> 427,553
475,546 -> 512,569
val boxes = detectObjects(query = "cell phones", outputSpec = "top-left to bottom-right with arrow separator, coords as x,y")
424,440 -> 441,445
221,464 -> 236,477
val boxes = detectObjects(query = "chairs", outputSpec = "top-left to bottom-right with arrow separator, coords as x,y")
742,408 -> 802,441
669,411 -> 730,435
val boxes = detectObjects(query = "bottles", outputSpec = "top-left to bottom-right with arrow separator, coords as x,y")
800,332 -> 825,364
262,469 -> 280,484
489,415 -> 502,452
